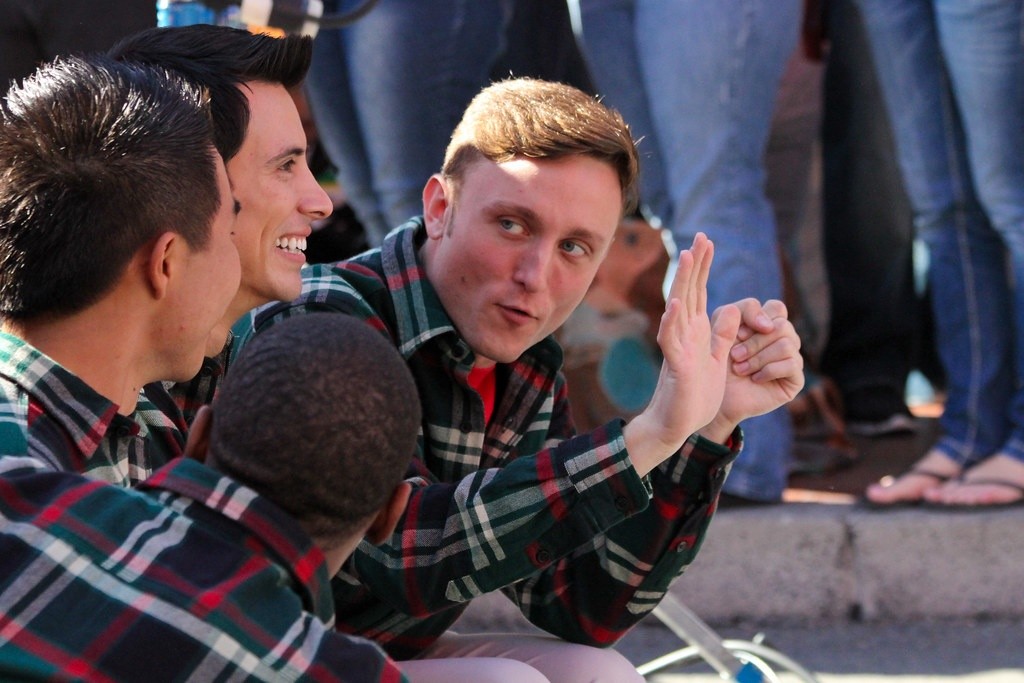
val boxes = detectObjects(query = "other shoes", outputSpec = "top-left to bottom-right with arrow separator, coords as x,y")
845,386 -> 918,436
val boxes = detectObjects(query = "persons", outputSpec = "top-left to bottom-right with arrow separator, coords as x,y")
303,0 -> 514,252
106,24 -> 334,487
0,52 -> 241,489
856,0 -> 1024,508
1,0 -> 955,476
0,309 -> 420,683
578,0 -> 806,507
231,77 -> 810,683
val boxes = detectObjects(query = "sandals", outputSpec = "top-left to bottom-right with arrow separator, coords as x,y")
919,474 -> 1024,511
861,463 -> 960,511
